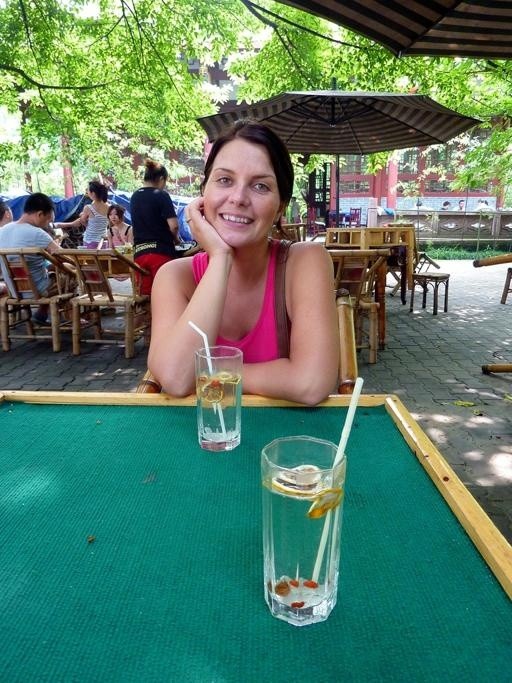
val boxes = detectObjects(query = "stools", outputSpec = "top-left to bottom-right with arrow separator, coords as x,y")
500,267 -> 512,306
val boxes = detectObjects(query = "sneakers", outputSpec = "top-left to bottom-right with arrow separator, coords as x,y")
30,314 -> 50,326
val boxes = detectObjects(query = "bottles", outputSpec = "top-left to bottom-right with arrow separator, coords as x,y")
367,197 -> 378,227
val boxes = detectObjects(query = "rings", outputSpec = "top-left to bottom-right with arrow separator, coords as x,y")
184,216 -> 192,222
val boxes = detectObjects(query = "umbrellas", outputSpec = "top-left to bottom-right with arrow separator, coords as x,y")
276,0 -> 512,61
195,88 -> 486,241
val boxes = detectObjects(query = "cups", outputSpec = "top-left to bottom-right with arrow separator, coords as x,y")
260,435 -> 347,628
194,345 -> 244,452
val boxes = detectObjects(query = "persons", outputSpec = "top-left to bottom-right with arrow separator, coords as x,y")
453,198 -> 465,210
410,200 -> 426,209
145,116 -> 343,407
129,157 -> 182,349
1,181 -> 134,331
439,200 -> 451,210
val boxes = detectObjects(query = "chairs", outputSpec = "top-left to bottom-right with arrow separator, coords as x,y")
134,292 -> 364,400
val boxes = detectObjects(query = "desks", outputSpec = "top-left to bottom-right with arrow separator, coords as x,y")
1,392 -> 511,682
